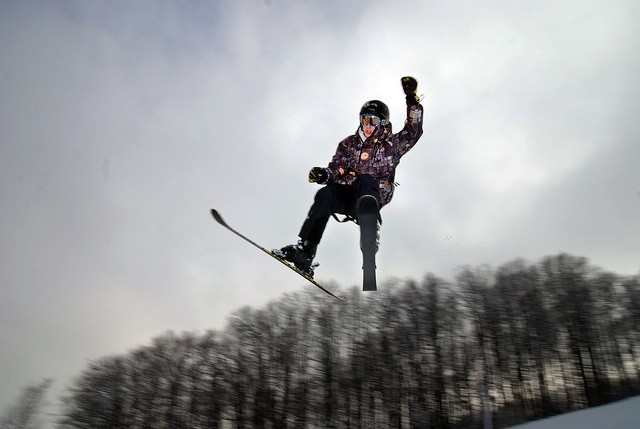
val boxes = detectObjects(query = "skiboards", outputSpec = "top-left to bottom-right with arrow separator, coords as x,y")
210,195 -> 379,300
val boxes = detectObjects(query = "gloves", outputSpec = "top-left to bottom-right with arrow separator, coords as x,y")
401,75 -> 420,104
308,167 -> 329,183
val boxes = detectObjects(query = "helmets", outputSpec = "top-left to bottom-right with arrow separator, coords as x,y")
359,100 -> 390,124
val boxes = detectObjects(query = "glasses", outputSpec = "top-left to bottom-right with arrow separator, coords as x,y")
362,114 -> 389,127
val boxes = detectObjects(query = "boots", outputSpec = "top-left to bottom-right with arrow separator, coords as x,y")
360,224 -> 380,253
280,242 -> 316,268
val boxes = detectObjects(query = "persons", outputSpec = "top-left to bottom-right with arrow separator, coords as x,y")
281,76 -> 423,273
